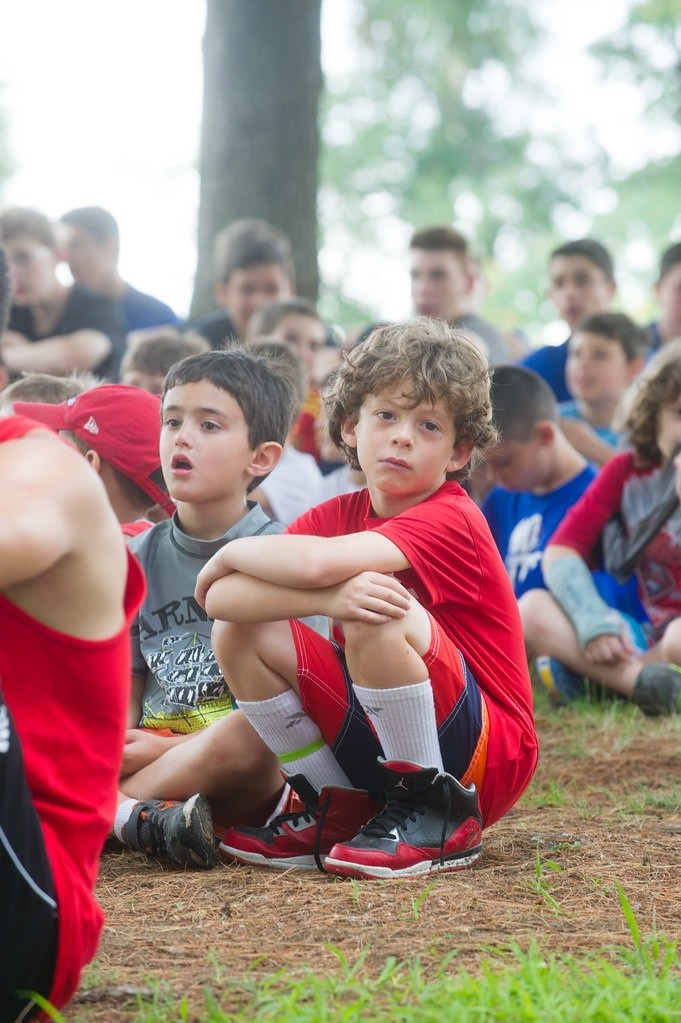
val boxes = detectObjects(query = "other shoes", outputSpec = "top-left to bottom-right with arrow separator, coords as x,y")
534,655 -> 585,707
630,664 -> 681,718
100,835 -> 123,855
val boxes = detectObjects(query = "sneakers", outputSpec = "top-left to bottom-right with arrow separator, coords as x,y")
120,793 -> 215,869
217,768 -> 378,871
322,755 -> 484,881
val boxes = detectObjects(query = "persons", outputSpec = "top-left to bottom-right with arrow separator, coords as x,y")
473,364 -> 649,625
0,251 -> 149,1023
0,206 -> 681,541
194,321 -> 539,881
518,337 -> 681,716
108,348 -> 330,872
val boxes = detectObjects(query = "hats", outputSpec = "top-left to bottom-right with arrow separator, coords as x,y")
13,384 -> 177,516
